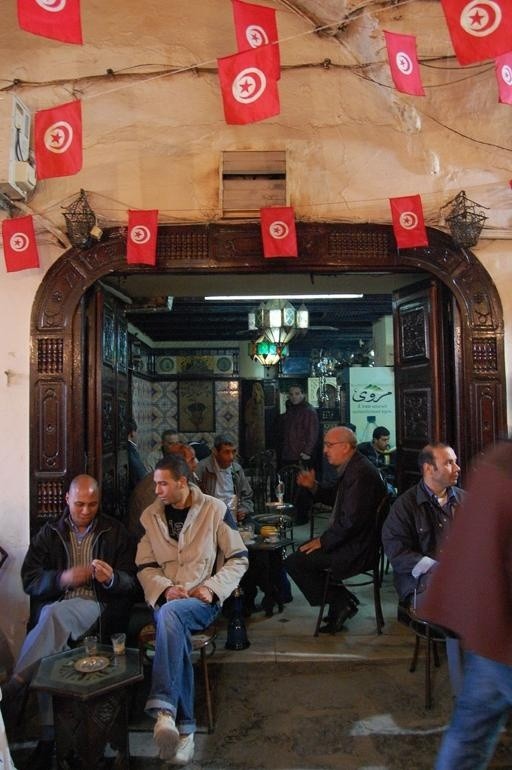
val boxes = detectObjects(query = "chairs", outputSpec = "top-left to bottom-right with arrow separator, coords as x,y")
314,492 -> 395,636
395,575 -> 457,708
140,557 -> 221,735
228,464 -> 302,620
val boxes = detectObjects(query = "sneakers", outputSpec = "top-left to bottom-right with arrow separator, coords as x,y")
167,733 -> 196,766
154,709 -> 180,760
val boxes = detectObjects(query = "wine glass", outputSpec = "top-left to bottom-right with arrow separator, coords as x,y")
83,635 -> 98,666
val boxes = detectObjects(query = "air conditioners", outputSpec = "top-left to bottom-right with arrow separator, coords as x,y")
0,92 -> 38,202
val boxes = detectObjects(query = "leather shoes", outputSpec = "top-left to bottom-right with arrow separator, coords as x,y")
27,743 -> 52,770
322,590 -> 360,624
320,594 -> 359,634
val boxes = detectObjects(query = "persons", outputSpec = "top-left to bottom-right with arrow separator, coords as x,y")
284,427 -> 389,633
269,383 -> 320,526
129,419 -> 258,630
414,441 -> 512,770
315,422 -> 389,507
381,441 -> 468,612
134,453 -> 250,766
0,473 -> 139,770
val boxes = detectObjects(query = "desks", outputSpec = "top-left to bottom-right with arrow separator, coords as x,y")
28,643 -> 146,770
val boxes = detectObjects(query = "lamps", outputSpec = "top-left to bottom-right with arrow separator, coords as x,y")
60,188 -> 95,249
444,190 -> 489,250
249,299 -> 297,368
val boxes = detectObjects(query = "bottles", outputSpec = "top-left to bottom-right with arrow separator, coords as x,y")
360,415 -> 380,443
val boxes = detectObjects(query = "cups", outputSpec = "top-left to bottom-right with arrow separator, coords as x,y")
240,531 -> 254,544
111,632 -> 127,655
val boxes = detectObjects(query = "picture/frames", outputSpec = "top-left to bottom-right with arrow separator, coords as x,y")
176,380 -> 217,434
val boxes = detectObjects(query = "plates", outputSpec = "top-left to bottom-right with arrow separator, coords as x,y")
75,656 -> 109,674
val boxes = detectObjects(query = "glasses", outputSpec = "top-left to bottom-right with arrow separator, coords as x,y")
324,439 -> 344,447
